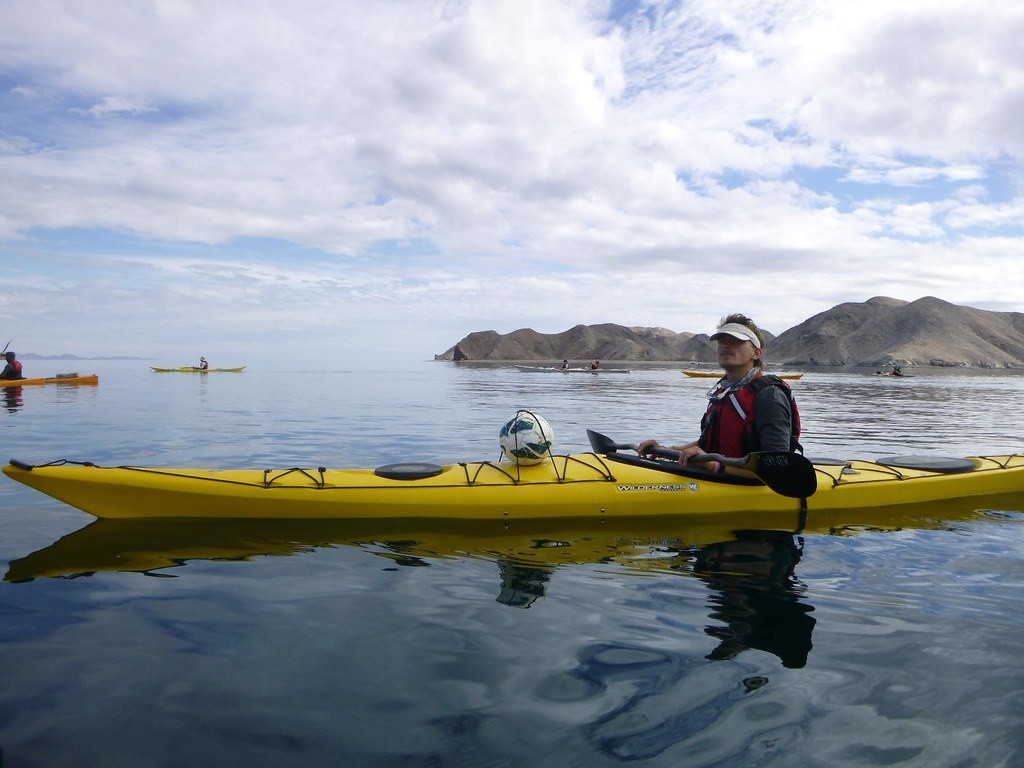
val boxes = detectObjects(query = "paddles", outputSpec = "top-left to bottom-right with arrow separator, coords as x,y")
585,428 -> 818,499
3,337 -> 15,353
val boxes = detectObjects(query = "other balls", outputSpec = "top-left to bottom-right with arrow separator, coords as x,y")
498,410 -> 555,467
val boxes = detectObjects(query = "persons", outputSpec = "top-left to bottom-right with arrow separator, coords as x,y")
893,365 -> 903,374
591,358 -> 599,369
563,360 -> 569,369
0,351 -> 23,379
199,356 -> 208,369
638,314 -> 801,479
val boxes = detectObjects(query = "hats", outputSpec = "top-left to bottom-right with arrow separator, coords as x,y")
709,323 -> 761,349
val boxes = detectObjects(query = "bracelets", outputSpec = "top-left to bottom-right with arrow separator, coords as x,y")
713,462 -> 725,474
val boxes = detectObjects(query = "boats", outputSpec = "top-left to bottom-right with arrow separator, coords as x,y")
150,366 -> 247,373
871,372 -> 916,377
0,455 -> 1023,524
0,502 -> 1024,582
515,365 -> 630,374
0,373 -> 99,387
681,369 -> 804,381
